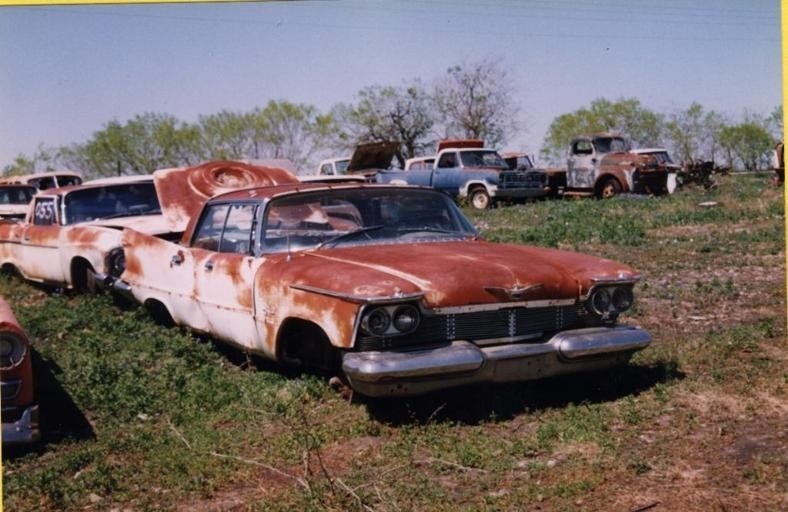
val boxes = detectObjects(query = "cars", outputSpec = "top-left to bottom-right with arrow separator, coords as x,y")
0,171 -> 164,296
122,158 -> 651,398
